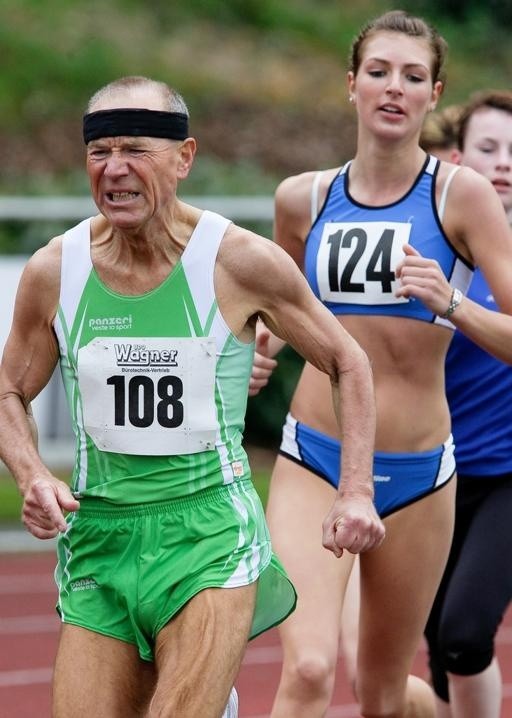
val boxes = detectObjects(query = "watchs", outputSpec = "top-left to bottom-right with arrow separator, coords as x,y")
442,285 -> 464,322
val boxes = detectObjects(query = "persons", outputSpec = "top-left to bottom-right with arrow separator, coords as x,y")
417,103 -> 469,166
250,8 -> 510,718
420,88 -> 511,718
1,73 -> 390,718
